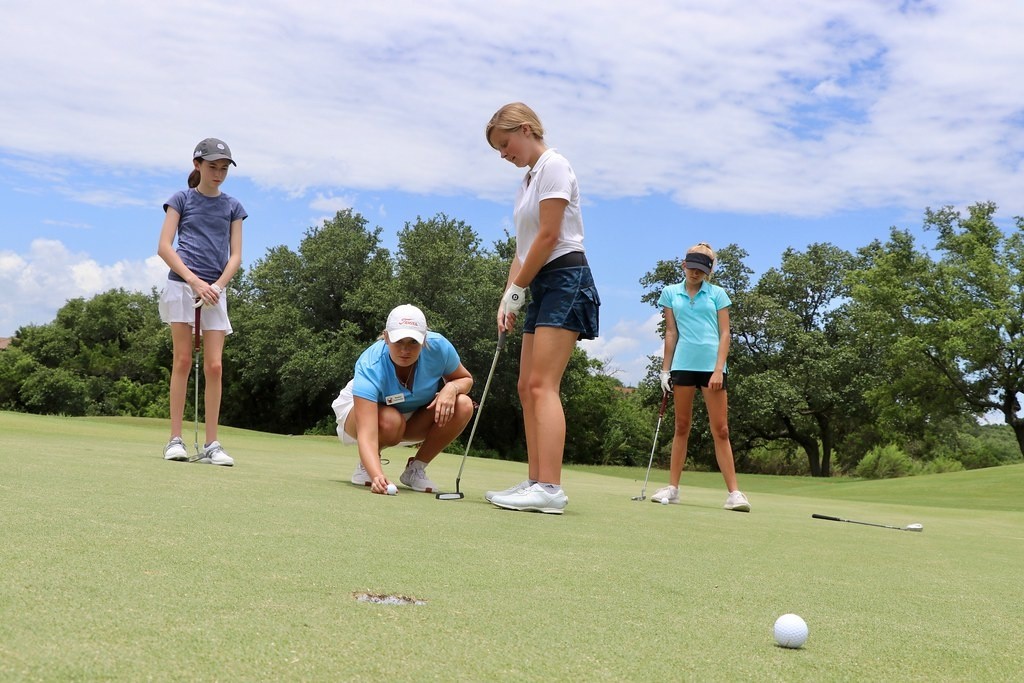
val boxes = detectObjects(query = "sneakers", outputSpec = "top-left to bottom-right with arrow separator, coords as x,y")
650,486 -> 680,504
163,437 -> 188,460
351,459 -> 390,486
399,457 -> 438,493
724,488 -> 751,512
203,441 -> 234,465
484,480 -> 568,514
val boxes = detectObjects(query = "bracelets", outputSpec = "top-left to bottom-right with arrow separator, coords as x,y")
448,382 -> 459,395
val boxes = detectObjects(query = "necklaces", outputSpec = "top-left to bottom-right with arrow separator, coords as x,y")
399,363 -> 414,390
196,187 -> 203,195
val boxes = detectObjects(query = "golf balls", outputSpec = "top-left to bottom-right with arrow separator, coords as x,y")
773,613 -> 809,648
662,498 -> 669,505
386,483 -> 397,495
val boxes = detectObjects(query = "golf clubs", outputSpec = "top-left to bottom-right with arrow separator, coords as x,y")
630,379 -> 671,502
811,512 -> 927,532
435,328 -> 507,500
188,297 -> 206,463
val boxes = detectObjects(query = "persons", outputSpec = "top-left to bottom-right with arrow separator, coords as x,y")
650,243 -> 751,512
158,138 -> 248,465
484,103 -> 599,514
332,304 -> 475,494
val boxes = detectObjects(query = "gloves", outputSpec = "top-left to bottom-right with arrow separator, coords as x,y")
502,283 -> 526,330
659,370 -> 674,398
192,284 -> 221,309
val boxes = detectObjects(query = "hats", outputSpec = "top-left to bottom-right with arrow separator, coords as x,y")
386,304 -> 427,345
193,138 -> 237,167
686,253 -> 714,276
